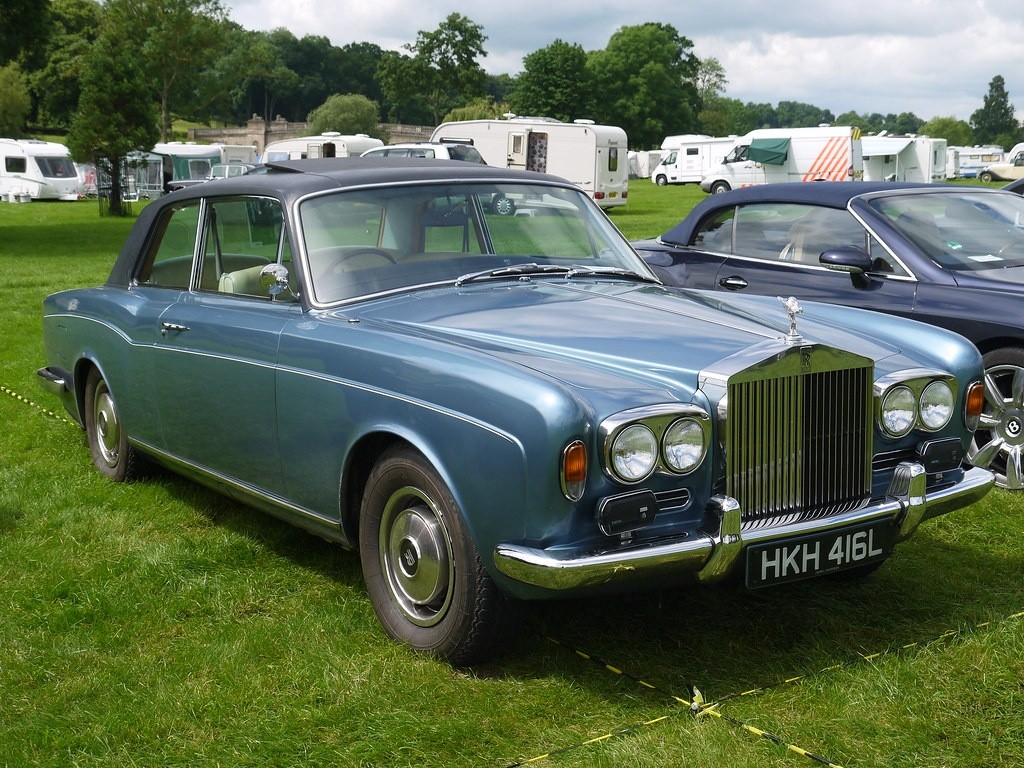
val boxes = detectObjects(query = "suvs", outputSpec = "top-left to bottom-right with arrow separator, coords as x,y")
341,138 -> 492,211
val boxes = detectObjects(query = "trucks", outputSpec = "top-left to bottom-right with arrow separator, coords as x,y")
861,129 -> 1004,184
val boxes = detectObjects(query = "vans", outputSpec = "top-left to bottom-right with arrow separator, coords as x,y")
700,123 -> 863,195
259,131 -> 385,158
428,109 -> 629,216
651,135 -> 742,186
978,142 -> 1024,184
0,138 -> 258,199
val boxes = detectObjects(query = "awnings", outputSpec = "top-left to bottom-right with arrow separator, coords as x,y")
746,138 -> 791,166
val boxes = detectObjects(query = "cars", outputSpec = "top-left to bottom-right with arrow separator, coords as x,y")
624,181 -> 1024,492
37,160 -> 995,669
166,163 -> 260,194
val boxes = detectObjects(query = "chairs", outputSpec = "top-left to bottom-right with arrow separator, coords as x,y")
219,260 -> 299,302
897,211 -> 940,256
784,220 -> 847,266
710,222 -> 767,258
